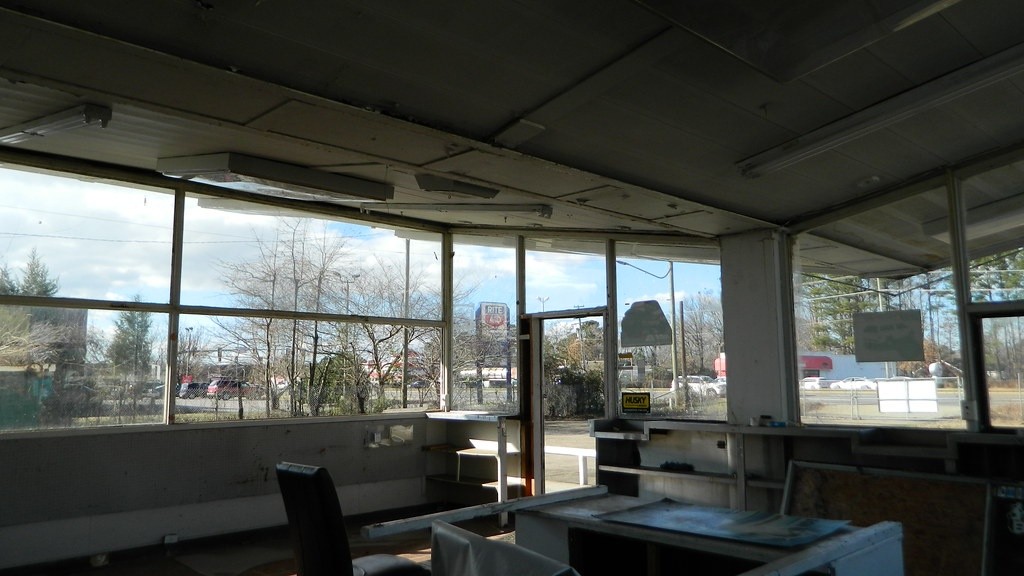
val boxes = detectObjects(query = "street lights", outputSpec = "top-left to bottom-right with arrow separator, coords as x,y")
333,271 -> 360,351
616,259 -> 677,380
184,327 -> 193,375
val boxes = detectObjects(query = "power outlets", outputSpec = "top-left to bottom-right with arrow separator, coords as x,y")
371,432 -> 382,443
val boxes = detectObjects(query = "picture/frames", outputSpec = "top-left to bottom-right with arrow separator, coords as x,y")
777,460 -> 991,575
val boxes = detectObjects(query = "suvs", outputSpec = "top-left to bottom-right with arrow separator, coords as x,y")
208,380 -> 264,401
670,374 -> 726,398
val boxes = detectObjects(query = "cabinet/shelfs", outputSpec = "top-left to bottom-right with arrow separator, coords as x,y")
586,413 -> 1024,575
424,409 -> 524,527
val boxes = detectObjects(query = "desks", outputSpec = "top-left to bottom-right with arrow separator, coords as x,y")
512,492 -> 905,576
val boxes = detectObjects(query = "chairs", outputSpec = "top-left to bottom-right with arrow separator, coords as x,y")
433,518 -> 584,576
274,462 -> 430,576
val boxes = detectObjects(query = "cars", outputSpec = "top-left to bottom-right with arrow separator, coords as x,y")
798,376 -> 828,390
413,380 -> 431,388
92,376 -> 210,400
830,376 -> 876,392
